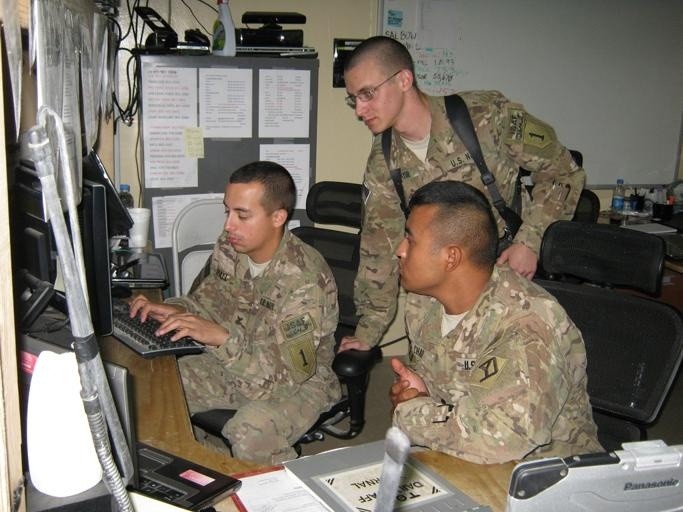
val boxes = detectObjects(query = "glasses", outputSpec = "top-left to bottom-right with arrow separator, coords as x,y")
345,69 -> 403,108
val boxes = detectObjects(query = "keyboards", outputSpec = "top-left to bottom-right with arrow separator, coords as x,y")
112,296 -> 208,359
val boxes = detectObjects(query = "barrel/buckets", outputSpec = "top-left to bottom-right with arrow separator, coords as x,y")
211,0 -> 238,56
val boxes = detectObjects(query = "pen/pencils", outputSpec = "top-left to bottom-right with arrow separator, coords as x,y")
627,186 -> 646,197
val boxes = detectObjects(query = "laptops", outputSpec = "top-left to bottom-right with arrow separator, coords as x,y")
15,326 -> 244,510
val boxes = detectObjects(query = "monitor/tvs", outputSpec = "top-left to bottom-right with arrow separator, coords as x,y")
8,154 -> 115,344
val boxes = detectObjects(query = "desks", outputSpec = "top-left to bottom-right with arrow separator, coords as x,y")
0,287 -> 520,512
598,210 -> 683,273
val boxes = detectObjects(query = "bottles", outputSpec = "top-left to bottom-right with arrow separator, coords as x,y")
117,184 -> 133,207
611,180 -> 624,221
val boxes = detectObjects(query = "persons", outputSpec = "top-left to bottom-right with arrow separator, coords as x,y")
389,181 -> 607,464
336,36 -> 586,354
129,162 -> 342,465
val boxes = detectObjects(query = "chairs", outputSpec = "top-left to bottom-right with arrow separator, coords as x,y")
533,220 -> 683,452
191,181 -> 384,458
569,150 -> 600,223
